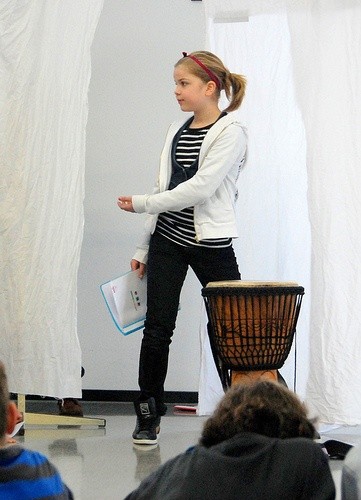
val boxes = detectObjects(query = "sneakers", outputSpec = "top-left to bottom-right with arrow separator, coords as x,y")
130,397 -> 162,446
56,397 -> 82,417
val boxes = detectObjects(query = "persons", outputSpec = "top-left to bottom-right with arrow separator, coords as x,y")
127,377 -> 336,500
116,50 -> 246,444
0,361 -> 76,500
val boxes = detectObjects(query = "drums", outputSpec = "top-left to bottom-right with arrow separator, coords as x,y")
201,281 -> 305,393
